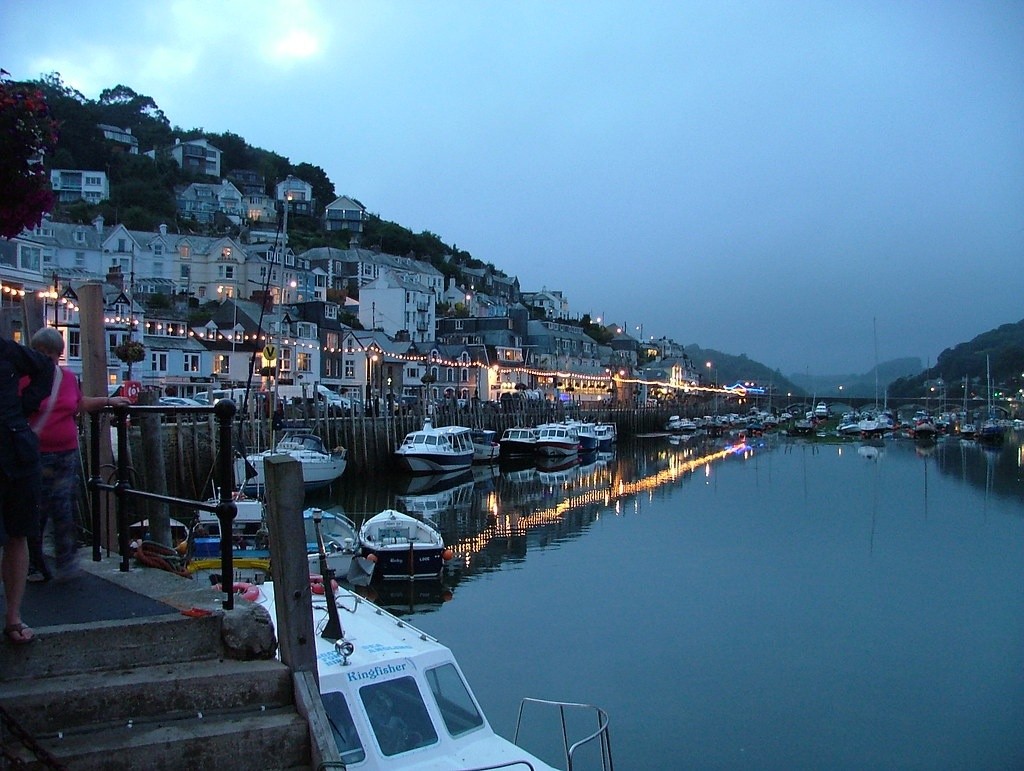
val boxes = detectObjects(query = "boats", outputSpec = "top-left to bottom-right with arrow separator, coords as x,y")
232,428 -> 349,497
393,417 -> 475,473
471,427 -> 501,460
500,417 -> 617,456
662,320 -> 1024,434
359,508 -> 451,579
123,486 -> 363,585
240,571 -> 563,771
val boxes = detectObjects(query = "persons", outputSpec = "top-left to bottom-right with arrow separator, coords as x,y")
18,325 -> 131,586
0,334 -> 56,646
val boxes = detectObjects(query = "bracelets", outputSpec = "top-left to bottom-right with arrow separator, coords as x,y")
107,397 -> 110,406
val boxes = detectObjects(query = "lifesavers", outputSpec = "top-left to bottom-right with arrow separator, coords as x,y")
213,581 -> 260,601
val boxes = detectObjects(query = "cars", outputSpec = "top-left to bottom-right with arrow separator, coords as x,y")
104,382 -> 485,415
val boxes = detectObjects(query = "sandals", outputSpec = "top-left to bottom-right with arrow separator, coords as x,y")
3,622 -> 37,644
